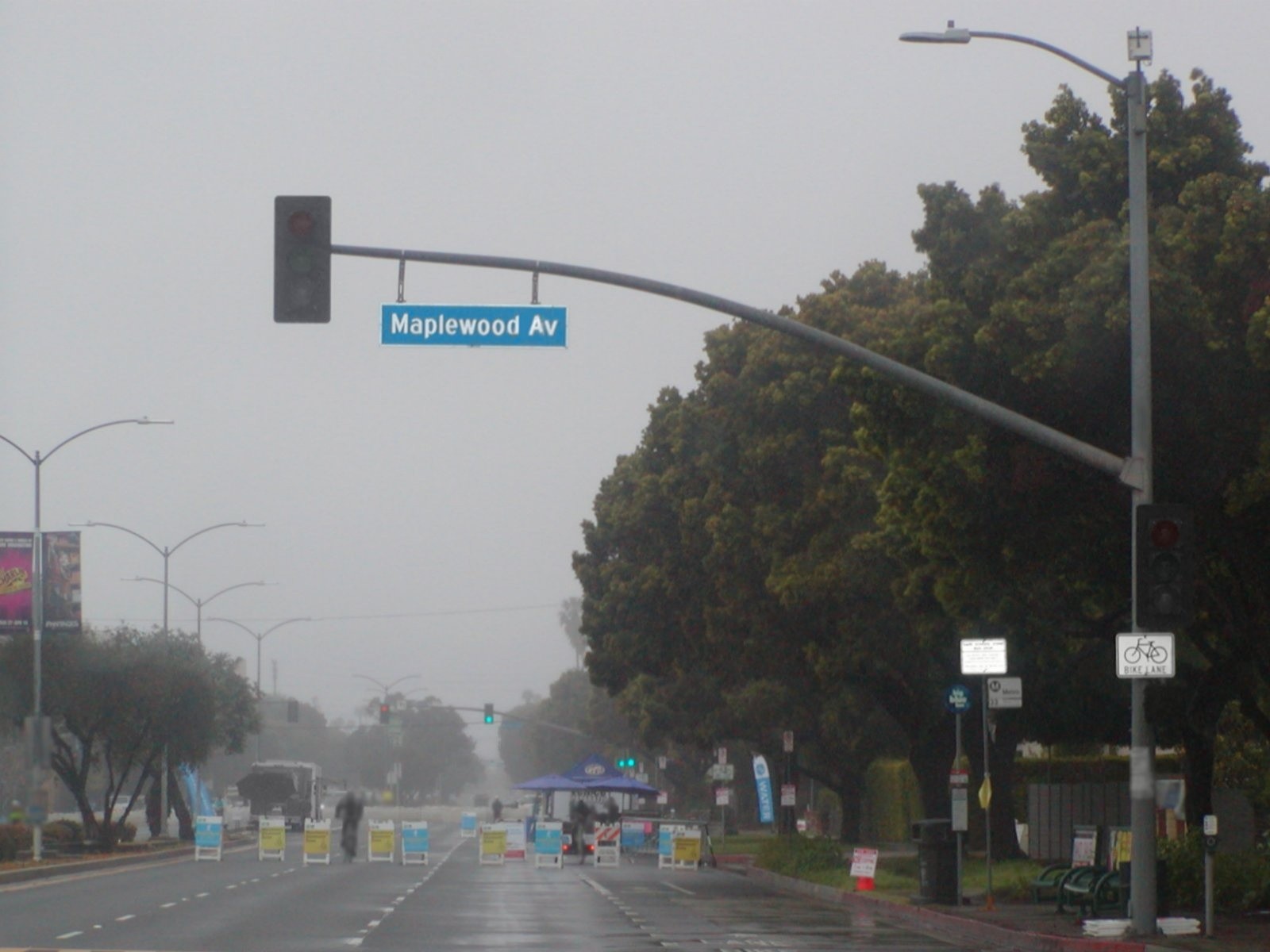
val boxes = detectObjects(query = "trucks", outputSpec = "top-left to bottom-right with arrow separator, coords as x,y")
243,752 -> 321,832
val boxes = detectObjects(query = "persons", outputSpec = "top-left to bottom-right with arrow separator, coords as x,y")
333,788 -> 364,856
493,798 -> 503,821
605,797 -> 619,821
574,800 -> 588,821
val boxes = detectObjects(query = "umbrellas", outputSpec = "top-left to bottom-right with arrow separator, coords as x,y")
587,776 -> 663,810
511,773 -> 586,815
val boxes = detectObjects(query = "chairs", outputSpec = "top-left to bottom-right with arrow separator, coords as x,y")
1029,820 -> 1107,914
1064,824 -> 1133,923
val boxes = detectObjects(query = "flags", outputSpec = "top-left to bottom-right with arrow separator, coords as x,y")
752,755 -> 774,823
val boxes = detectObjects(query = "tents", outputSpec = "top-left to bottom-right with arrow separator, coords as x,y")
562,751 -> 623,782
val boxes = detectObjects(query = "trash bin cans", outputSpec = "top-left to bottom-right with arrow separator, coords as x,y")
909,818 -> 972,908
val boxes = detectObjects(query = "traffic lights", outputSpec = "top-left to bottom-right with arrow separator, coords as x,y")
272,191 -> 336,325
485,703 -> 494,726
618,757 -> 636,768
378,703 -> 392,724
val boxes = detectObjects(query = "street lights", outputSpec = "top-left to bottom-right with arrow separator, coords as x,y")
0,412 -> 175,865
207,612 -> 312,696
136,571 -> 274,653
72,509 -> 270,839
904,20 -> 1161,942
